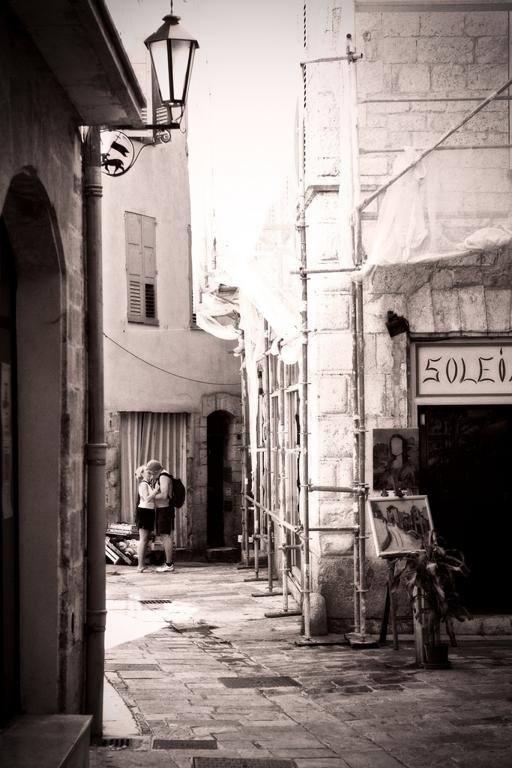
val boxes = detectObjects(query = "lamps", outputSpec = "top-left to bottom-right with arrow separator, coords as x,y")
87,0 -> 200,177
386,311 -> 410,338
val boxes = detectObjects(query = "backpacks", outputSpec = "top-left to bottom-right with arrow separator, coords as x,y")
159,473 -> 185,508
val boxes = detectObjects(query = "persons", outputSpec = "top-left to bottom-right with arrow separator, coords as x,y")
378,431 -> 418,492
143,459 -> 178,574
134,465 -> 160,574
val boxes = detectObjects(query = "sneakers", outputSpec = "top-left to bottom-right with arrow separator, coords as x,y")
137,563 -> 175,573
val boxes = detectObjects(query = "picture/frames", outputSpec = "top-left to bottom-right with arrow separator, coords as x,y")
366,493 -> 441,558
